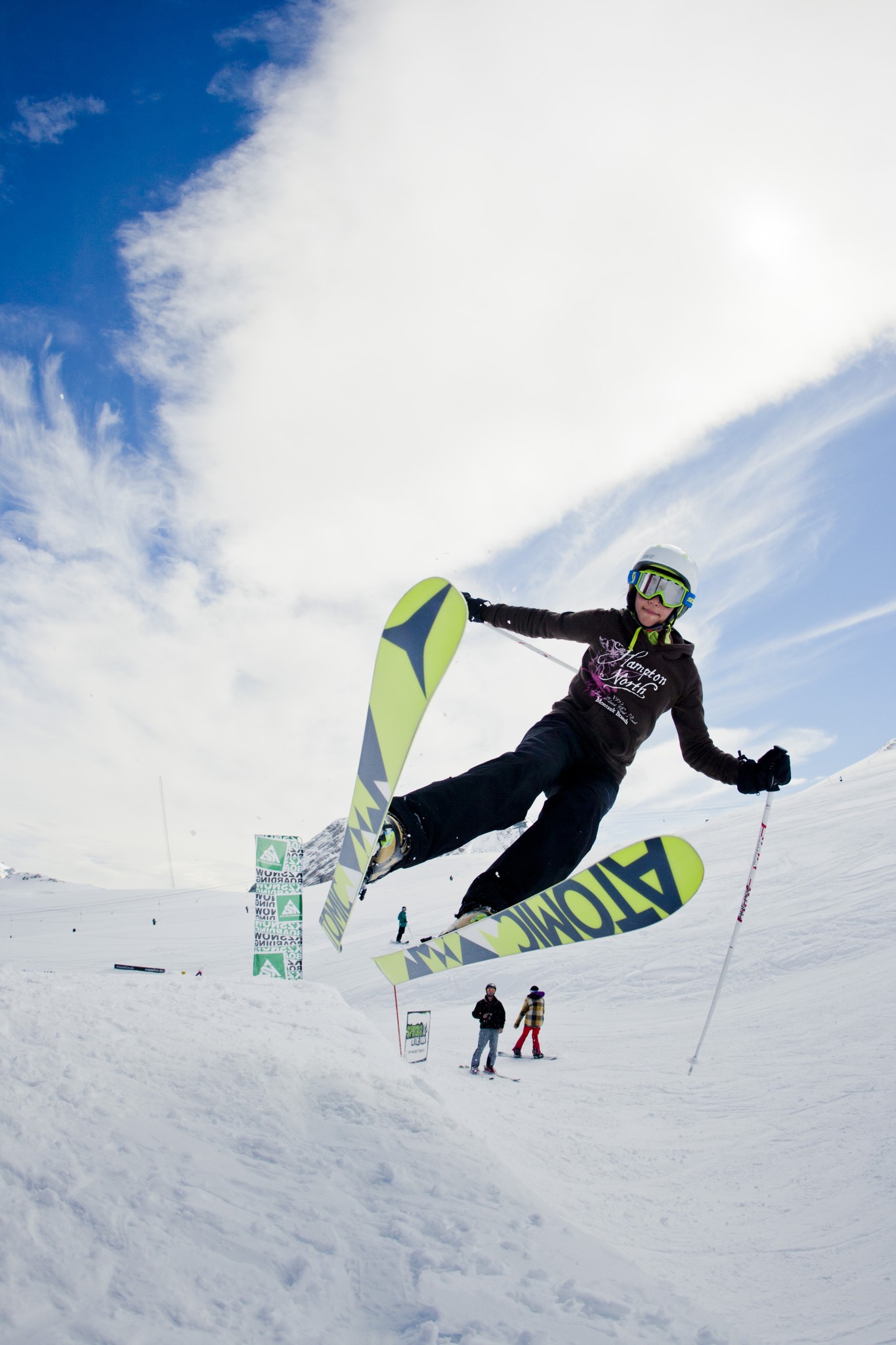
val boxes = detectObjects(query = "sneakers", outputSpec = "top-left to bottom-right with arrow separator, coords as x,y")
396,941 -> 400,943
514,1048 -> 521,1057
533,1049 -> 544,1058
398,940 -> 401,942
358,812 -> 410,897
439,910 -> 492,937
484,1066 -> 496,1072
471,1067 -> 480,1074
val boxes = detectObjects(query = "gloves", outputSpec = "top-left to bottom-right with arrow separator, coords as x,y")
460,592 -> 491,624
737,749 -> 792,795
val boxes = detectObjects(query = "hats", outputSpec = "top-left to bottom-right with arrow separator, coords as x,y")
530,986 -> 539,992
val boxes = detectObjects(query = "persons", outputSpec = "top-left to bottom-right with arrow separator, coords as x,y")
245,906 -> 249,913
470,983 -> 506,1075
511,985 -> 545,1059
195,967 -> 204,976
364,540 -> 793,939
152,918 -> 156,925
396,906 -> 408,943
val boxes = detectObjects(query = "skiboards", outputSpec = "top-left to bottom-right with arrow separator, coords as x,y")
460,1065 -> 521,1081
319,575 -> 704,986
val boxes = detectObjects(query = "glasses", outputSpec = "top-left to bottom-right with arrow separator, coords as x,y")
627,570 -> 689,607
487,989 -> 495,992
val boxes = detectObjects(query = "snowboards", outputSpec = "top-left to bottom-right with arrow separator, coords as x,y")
498,1052 -> 556,1060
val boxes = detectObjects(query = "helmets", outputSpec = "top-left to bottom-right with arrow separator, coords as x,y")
402,907 -> 406,909
630,543 -> 699,596
485,983 -> 497,990
